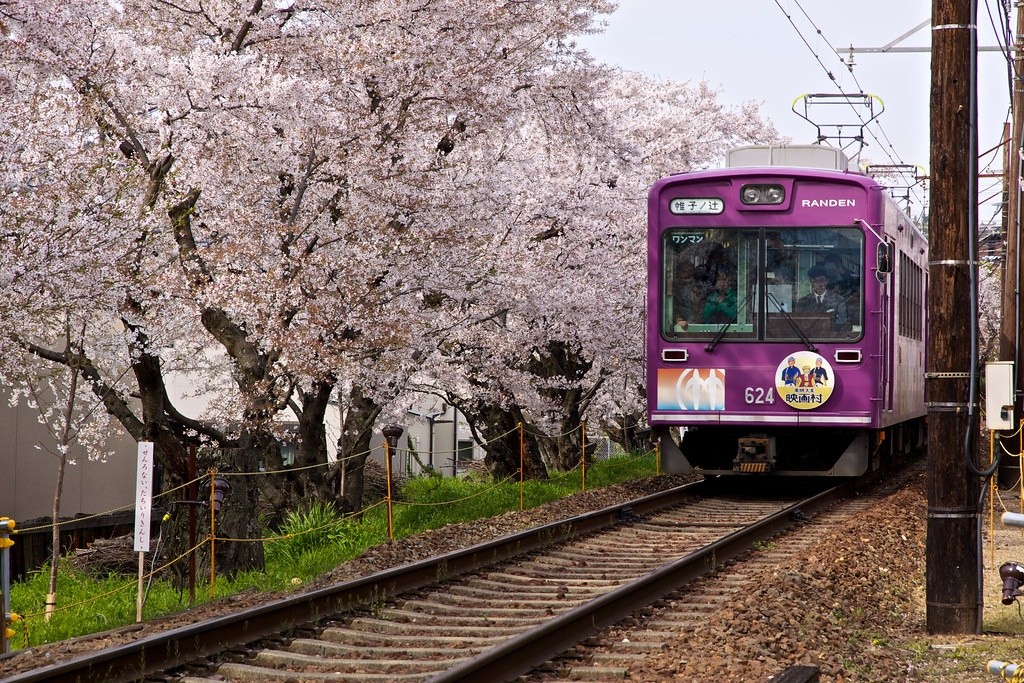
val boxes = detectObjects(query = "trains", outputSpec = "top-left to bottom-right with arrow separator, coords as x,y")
646,145 -> 930,486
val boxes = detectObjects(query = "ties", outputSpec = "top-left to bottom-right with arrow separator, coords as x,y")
817,296 -> 822,309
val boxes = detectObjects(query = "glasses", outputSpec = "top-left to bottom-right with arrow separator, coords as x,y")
811,278 -> 827,283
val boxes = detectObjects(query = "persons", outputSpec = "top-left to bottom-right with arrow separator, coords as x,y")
703,267 -> 737,323
673,265 -> 707,331
796,266 -> 848,324
